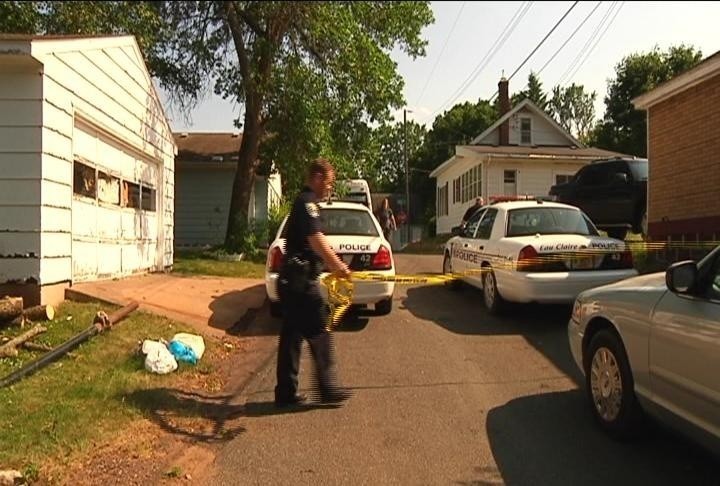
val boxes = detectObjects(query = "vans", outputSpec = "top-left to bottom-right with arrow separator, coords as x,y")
324,179 -> 374,212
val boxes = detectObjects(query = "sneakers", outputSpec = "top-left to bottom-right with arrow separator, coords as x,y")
275,388 -> 356,404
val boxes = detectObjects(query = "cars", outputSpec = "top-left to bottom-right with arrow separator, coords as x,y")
549,156 -> 648,240
565,245 -> 720,448
264,200 -> 395,314
442,198 -> 640,315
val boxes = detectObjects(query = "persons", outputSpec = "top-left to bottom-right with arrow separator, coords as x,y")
270,156 -> 356,413
460,195 -> 484,231
395,210 -> 408,228
375,197 -> 398,243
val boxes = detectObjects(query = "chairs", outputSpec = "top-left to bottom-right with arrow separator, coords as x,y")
345,218 -> 360,230
